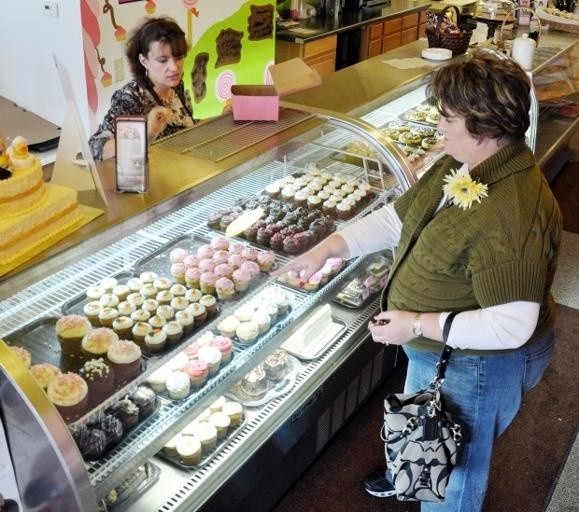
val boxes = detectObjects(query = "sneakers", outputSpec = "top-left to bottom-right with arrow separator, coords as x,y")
362,468 -> 397,498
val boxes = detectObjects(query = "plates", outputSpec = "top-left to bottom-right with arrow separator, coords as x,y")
423,47 -> 453,62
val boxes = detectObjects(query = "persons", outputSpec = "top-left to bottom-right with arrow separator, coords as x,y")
87,15 -> 197,160
267,47 -> 566,511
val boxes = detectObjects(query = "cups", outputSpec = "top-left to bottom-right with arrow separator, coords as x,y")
513,33 -> 536,70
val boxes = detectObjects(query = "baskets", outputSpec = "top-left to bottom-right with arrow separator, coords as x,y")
498,7 -> 542,57
536,6 -> 579,34
426,5 -> 473,55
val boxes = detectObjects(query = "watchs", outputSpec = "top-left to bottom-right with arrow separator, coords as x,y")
413,312 -> 423,339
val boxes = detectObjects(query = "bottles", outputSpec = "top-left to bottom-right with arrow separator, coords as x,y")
299,4 -> 317,19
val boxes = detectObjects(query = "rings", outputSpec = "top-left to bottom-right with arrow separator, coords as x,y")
385,340 -> 389,347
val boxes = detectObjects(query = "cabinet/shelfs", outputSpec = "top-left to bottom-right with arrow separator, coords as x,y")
275,35 -> 338,85
359,10 -> 427,62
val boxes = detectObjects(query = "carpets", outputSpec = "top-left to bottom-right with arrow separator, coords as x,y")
261,161 -> 579,509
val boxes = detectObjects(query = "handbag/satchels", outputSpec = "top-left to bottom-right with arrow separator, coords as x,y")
380,383 -> 464,502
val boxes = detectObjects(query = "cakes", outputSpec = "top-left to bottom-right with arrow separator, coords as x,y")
265,165 -> 372,218
146,331 -> 234,400
294,303 -> 333,348
84,270 -> 219,353
170,236 -> 275,299
216,285 -> 290,344
242,347 -> 288,397
278,253 -> 347,289
71,387 -> 156,461
345,98 -> 443,166
0,130 -> 86,267
8,314 -> 143,419
163,395 -> 244,464
209,193 -> 334,254
334,256 -> 392,304
